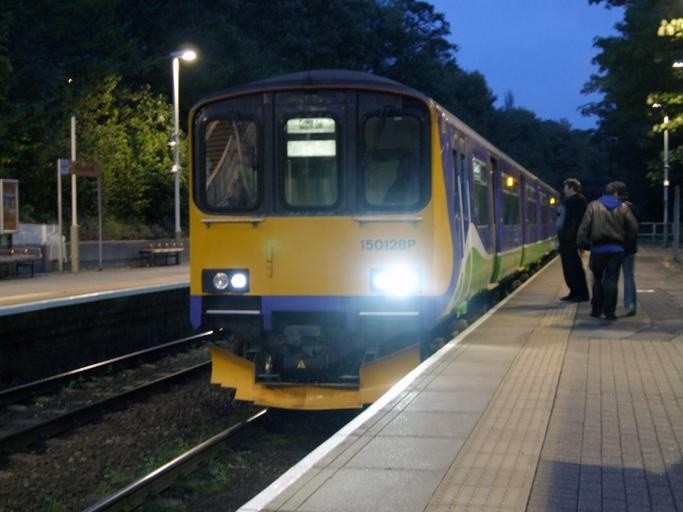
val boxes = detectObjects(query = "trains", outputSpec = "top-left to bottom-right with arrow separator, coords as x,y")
185,67 -> 565,414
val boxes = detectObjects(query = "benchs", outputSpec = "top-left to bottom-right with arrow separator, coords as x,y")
0,246 -> 42,278
139,246 -> 183,266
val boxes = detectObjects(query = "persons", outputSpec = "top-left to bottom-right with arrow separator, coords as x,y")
555,179 -> 590,302
575,183 -> 638,319
612,181 -> 638,317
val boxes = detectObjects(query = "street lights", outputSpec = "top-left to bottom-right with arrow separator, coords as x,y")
672,60 -> 683,264
166,42 -> 199,243
652,102 -> 669,244
67,77 -> 82,273
